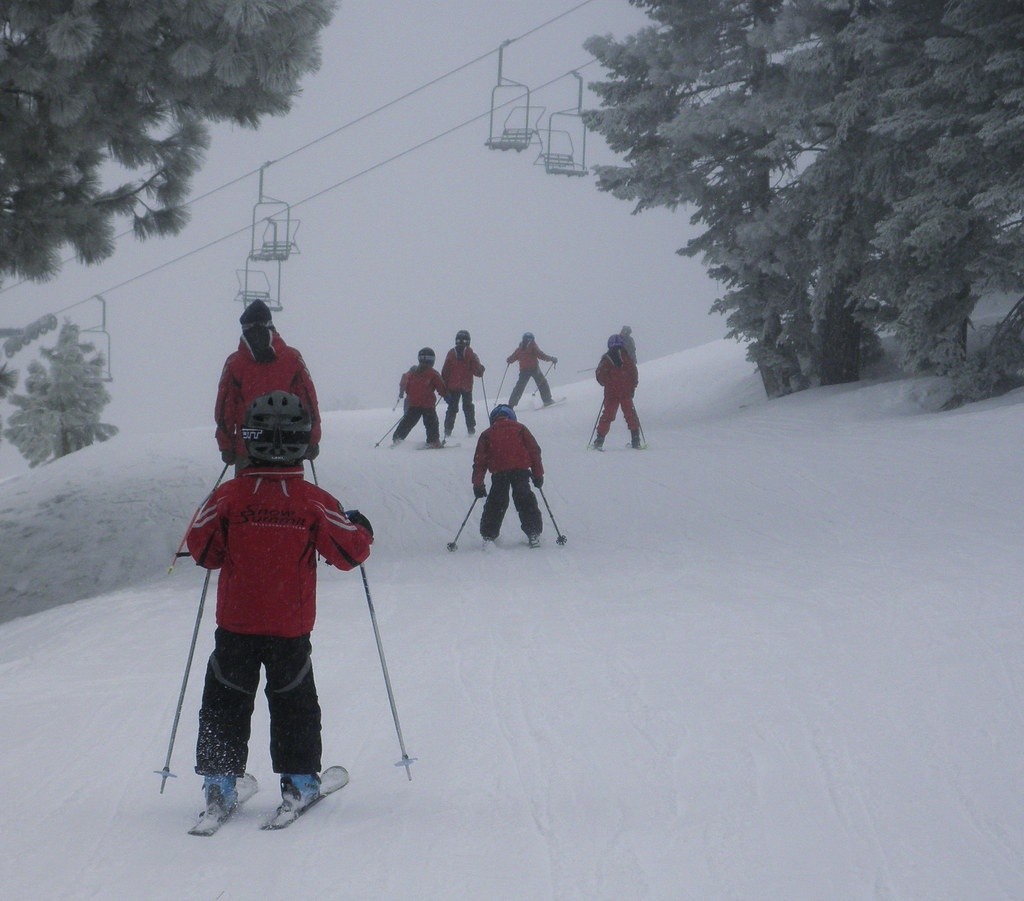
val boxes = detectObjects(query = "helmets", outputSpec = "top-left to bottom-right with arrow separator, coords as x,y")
608,334 -> 625,348
522,332 -> 535,339
242,390 -> 312,465
490,404 -> 517,421
418,347 -> 435,360
455,330 -> 471,345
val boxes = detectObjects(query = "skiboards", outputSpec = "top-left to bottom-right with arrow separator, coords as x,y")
389,441 -> 461,450
513,396 -> 568,412
586,442 -> 649,452
482,537 -> 543,552
186,762 -> 353,837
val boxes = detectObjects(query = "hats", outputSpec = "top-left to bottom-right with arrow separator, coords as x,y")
240,299 -> 275,332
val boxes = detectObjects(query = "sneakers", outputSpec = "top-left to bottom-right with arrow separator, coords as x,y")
425,440 -> 442,448
544,400 -> 555,406
529,534 -> 540,547
280,772 -> 322,810
594,434 -> 605,448
201,772 -> 239,820
632,430 -> 641,448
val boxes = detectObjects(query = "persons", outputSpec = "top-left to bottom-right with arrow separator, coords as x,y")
215,299 -> 322,481
187,391 -> 374,817
471,404 -> 544,546
593,325 -> 642,450
506,332 -> 558,410
392,330 -> 486,449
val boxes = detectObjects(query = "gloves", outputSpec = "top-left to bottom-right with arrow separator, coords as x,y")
473,485 -> 487,498
222,450 -> 237,465
552,356 -> 558,363
533,476 -> 544,488
344,509 -> 374,536
306,444 -> 319,460
